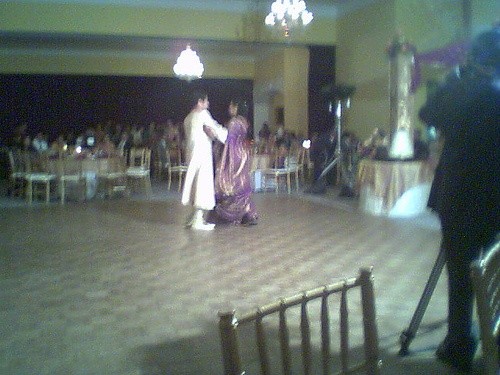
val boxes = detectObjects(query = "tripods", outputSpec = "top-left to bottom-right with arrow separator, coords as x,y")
314,100 -> 349,189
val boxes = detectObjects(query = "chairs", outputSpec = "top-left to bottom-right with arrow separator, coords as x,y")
154,142 -> 188,192
6,149 -> 57,204
216,240 -> 500,375
261,148 -> 314,198
126,146 -> 154,200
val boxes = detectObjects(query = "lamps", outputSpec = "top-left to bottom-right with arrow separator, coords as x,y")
265,0 -> 314,38
173,45 -> 205,83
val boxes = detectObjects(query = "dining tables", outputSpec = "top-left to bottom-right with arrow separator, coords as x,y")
357,156 -> 434,217
212,150 -> 271,169
48,152 -> 127,202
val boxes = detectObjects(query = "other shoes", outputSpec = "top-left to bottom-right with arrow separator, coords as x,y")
242,216 -> 259,225
190,217 -> 216,231
435,335 -> 478,368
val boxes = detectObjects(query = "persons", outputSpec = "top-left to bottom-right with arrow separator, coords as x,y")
202,98 -> 261,226
417,32 -> 500,361
179,89 -> 229,231
0,118 -> 431,188
